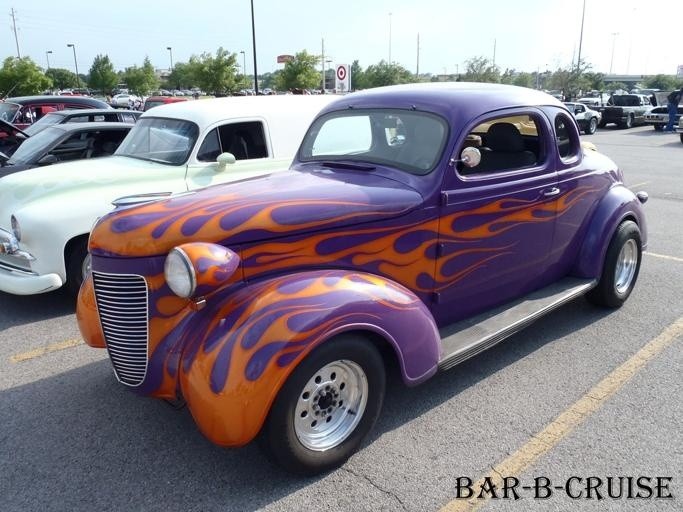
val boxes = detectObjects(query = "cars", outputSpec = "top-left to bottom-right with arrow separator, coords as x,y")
537,84 -> 683,146
75,82 -> 650,477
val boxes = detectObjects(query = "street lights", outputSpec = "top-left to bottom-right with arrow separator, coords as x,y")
67,44 -> 80,95
166,47 -> 174,97
240,51 -> 245,90
607,30 -> 617,74
45,51 -> 53,95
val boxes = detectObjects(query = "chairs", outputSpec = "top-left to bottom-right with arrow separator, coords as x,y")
460,121 -> 538,178
78,130 -> 102,160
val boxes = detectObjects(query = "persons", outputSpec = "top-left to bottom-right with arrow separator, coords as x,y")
663,87 -> 683,133
